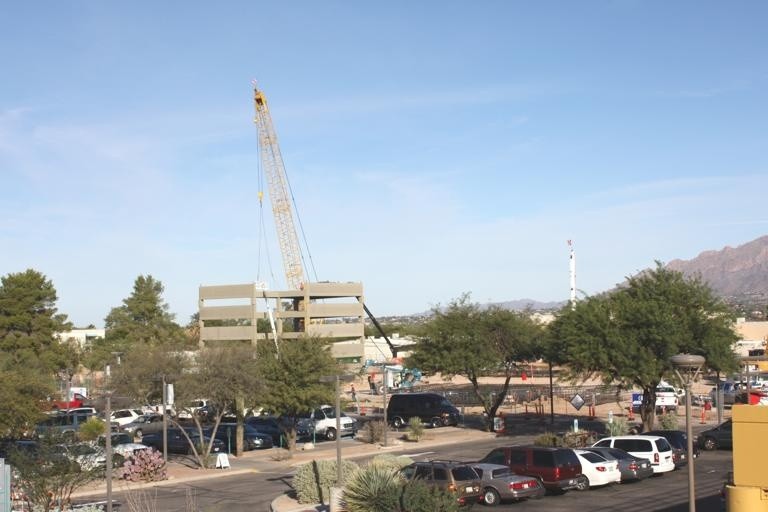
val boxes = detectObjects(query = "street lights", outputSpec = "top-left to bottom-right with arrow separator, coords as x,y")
669,351 -> 706,512
101,391 -> 113,512
317,374 -> 355,489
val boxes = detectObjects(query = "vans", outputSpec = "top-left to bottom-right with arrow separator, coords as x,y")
386,393 -> 460,428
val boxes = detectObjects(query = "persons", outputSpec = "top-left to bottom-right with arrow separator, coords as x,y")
350,382 -> 357,402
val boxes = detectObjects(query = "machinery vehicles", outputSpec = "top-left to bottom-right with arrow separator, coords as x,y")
357,359 -> 421,395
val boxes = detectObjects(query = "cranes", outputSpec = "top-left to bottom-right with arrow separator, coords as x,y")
249,77 -> 325,333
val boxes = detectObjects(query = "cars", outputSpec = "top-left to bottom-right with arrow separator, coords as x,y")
631,385 -> 686,413
695,418 -> 732,452
709,380 -> 752,403
